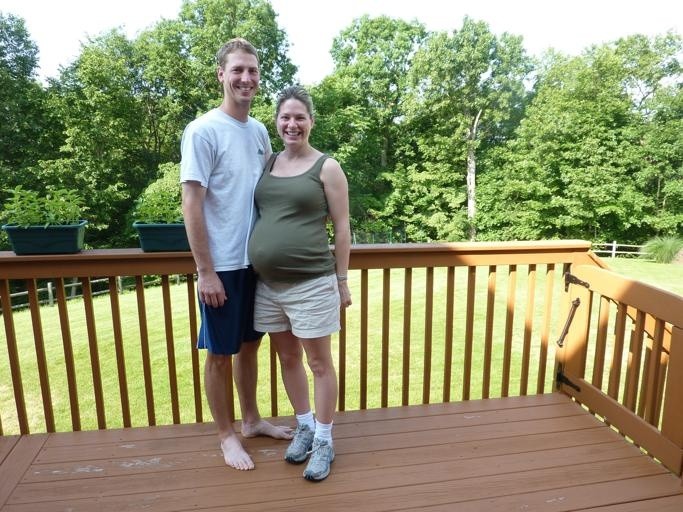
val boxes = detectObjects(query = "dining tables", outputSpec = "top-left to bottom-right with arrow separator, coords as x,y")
132,189 -> 191,252
0,183 -> 90,256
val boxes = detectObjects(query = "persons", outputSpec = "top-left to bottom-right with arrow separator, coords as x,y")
176,38 -> 295,475
243,83 -> 353,484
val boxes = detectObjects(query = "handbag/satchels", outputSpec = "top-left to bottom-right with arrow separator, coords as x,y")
337,275 -> 347,281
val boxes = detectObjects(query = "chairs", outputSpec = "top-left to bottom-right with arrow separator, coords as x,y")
283,424 -> 315,463
303,437 -> 334,481
